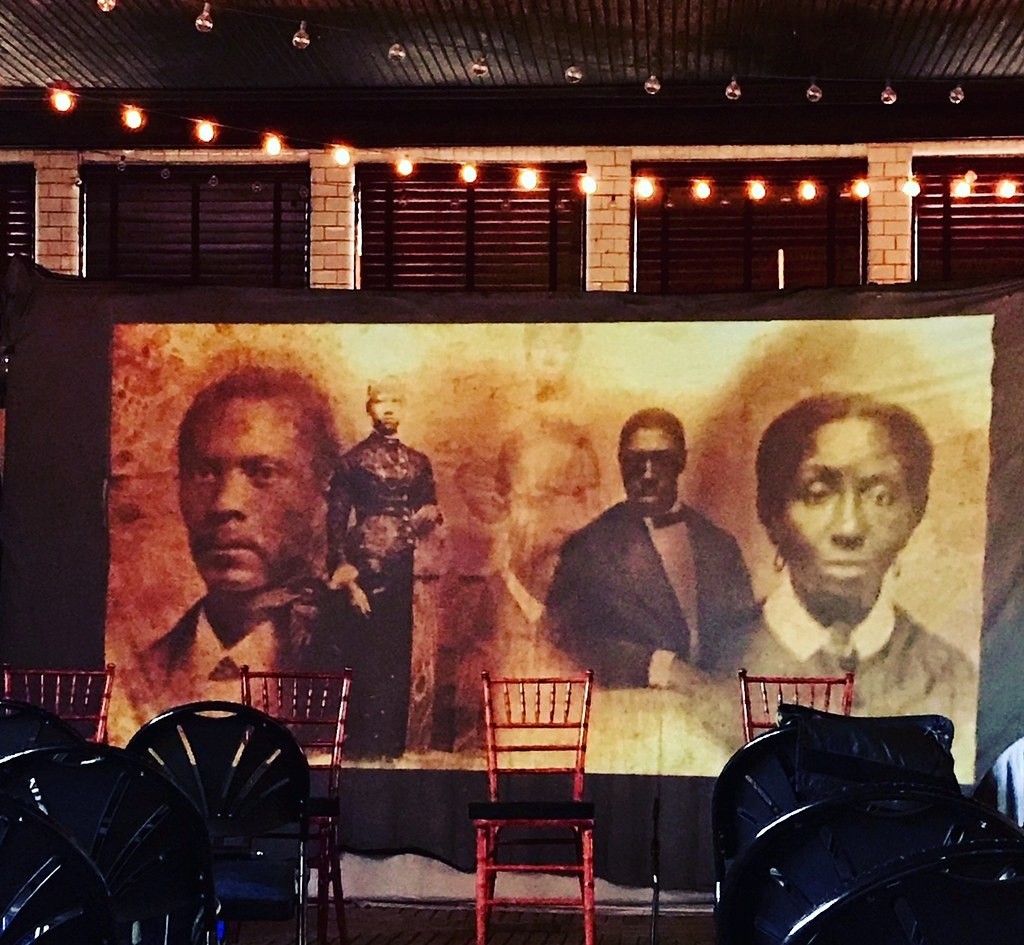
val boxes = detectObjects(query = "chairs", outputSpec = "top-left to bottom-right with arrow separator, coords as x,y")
713,666 -> 1023,945
0,664 -> 353,945
471,667 -> 601,945
